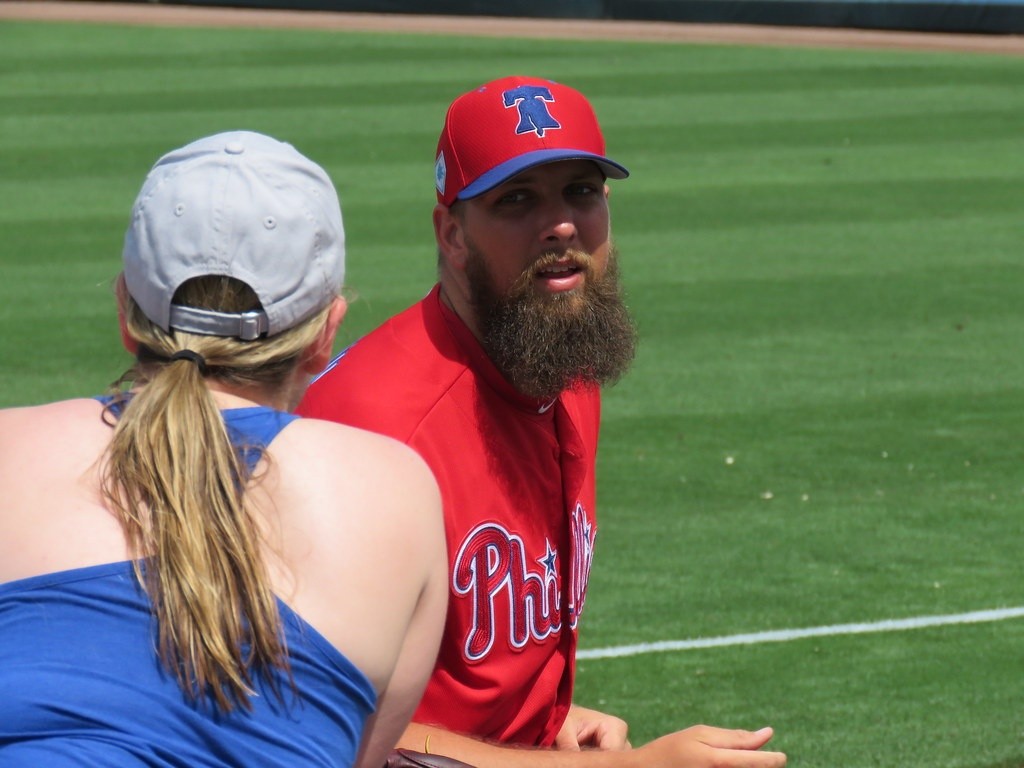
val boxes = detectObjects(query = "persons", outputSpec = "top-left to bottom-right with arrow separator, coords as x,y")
0,128 -> 449,768
292,77 -> 789,766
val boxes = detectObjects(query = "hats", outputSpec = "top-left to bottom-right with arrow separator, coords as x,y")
123,130 -> 344,340
435,75 -> 629,206
